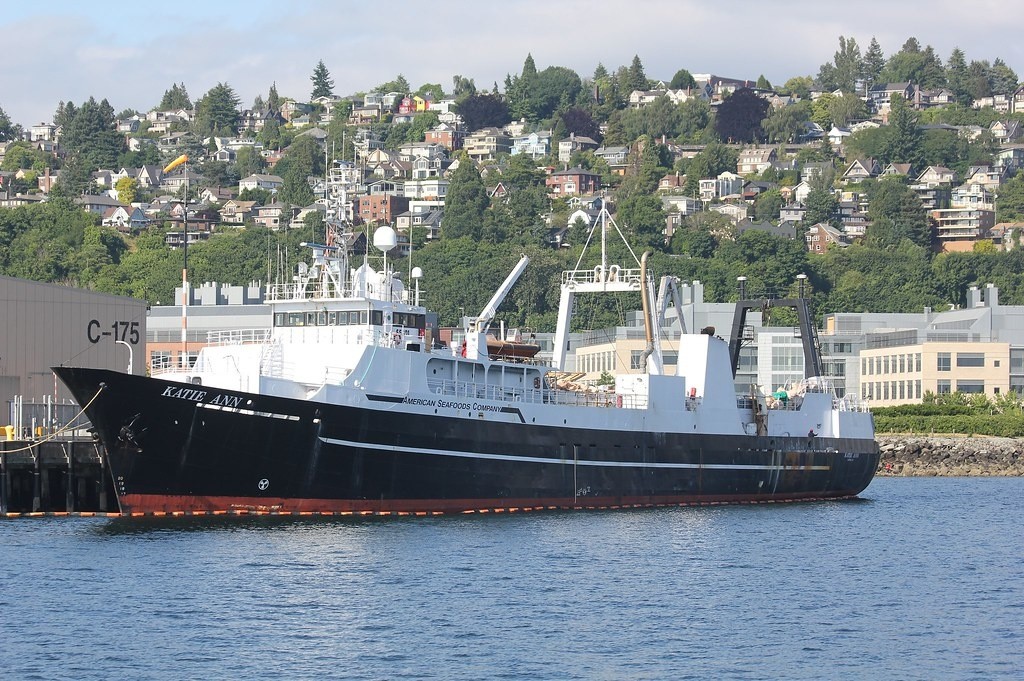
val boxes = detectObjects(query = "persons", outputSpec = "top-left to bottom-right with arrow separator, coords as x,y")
804,429 -> 819,453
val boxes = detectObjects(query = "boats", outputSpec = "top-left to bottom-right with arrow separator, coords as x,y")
47,193 -> 881,522
0,130 -> 624,519
